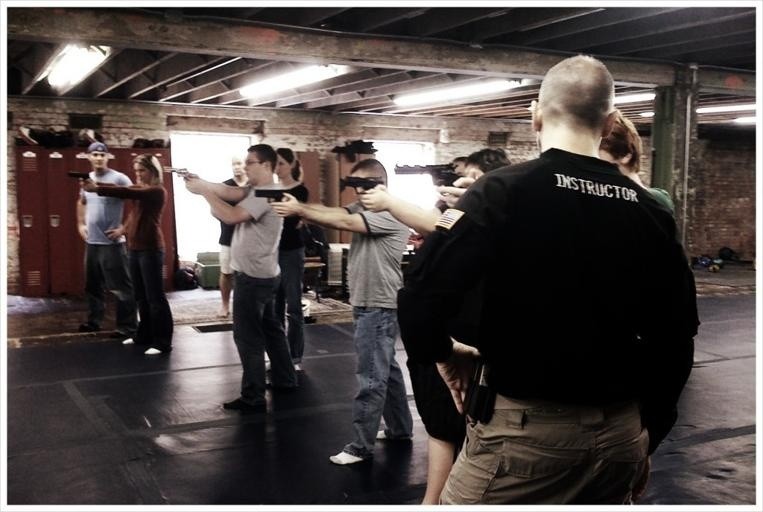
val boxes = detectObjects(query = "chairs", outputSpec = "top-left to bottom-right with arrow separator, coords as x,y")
196,252 -> 219,289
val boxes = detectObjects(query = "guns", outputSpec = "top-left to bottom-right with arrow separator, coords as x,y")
342,176 -> 383,192
396,164 -> 467,189
164,167 -> 191,178
254,189 -> 295,203
70,171 -> 89,181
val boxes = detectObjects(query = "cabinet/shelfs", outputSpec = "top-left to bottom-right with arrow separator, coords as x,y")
328,153 -> 375,243
14,145 -> 177,297
295,152 -> 321,223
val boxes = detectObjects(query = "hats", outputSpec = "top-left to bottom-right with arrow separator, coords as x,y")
89,142 -> 107,154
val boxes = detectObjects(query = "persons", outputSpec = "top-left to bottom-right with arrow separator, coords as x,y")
185,144 -> 298,411
361,149 -> 511,241
77,143 -> 135,338
272,147 -> 309,366
397,54 -> 701,505
216,154 -> 248,318
600,108 -> 674,216
269,159 -> 413,465
79,154 -> 173,357
